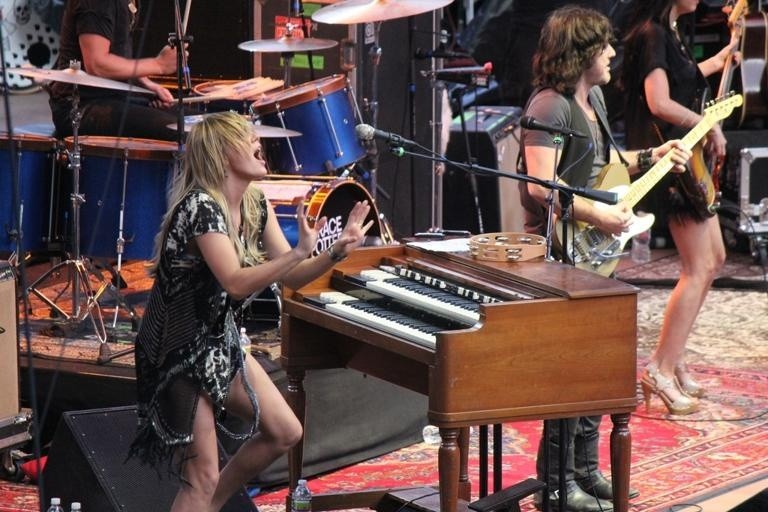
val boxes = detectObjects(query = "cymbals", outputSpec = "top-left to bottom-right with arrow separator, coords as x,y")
3,67 -> 159,94
238,39 -> 339,52
311,2 -> 455,25
166,122 -> 301,139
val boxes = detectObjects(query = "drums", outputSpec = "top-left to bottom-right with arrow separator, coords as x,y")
191,78 -> 292,112
251,72 -> 370,175
241,177 -> 381,256
59,135 -> 179,261
0,132 -> 58,263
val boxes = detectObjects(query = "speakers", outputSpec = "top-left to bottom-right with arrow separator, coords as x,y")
42,405 -> 256,512
443,105 -> 524,234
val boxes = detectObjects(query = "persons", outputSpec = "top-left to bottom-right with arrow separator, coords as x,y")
512,5 -> 693,511
117,108 -> 375,511
47,1 -> 193,143
609,0 -> 744,418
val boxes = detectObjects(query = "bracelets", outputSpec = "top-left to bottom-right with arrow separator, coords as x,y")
637,149 -> 653,173
326,245 -> 346,262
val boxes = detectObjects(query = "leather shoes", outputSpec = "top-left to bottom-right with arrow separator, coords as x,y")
533,480 -> 614,512
577,468 -> 640,502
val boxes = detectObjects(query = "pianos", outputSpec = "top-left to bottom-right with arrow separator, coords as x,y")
282,235 -> 640,512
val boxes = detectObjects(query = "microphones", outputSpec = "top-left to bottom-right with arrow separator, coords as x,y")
354,124 -> 417,148
417,62 -> 492,79
520,116 -> 588,140
415,46 -> 470,58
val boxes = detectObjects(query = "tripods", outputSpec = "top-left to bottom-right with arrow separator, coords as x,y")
18,85 -> 141,356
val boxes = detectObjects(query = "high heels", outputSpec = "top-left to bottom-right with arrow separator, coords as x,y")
675,360 -> 709,398
640,364 -> 702,415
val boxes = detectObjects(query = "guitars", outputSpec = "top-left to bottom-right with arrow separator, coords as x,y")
677,0 -> 749,220
551,88 -> 744,279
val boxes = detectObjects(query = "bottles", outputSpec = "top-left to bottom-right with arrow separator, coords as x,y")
630,210 -> 653,263
424,425 -> 474,444
45,496 -> 82,511
291,477 -> 311,512
